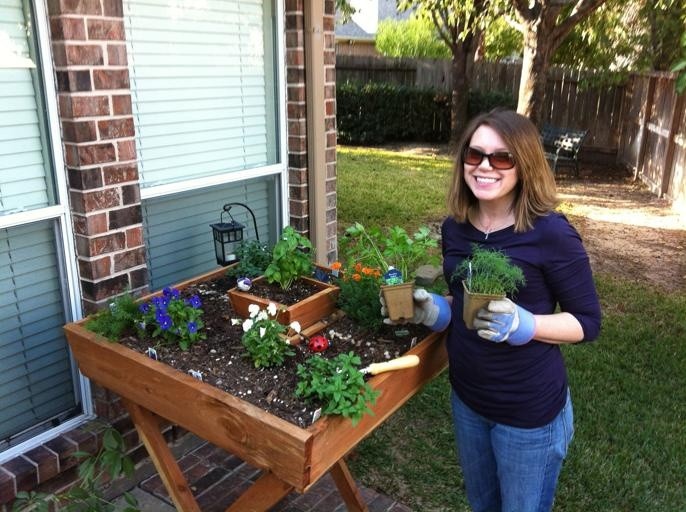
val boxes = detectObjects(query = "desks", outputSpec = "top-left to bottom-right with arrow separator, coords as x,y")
63,252 -> 452,512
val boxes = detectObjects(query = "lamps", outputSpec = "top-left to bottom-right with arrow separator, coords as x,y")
209,202 -> 263,266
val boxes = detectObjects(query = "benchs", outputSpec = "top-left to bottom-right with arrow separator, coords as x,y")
539,122 -> 590,178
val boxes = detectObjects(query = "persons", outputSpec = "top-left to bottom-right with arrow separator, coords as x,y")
377,106 -> 605,512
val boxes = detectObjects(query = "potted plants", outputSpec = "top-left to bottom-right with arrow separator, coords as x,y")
345,221 -> 438,321
227,226 -> 345,345
450,242 -> 527,331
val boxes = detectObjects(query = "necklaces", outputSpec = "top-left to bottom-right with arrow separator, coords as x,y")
475,208 -> 508,240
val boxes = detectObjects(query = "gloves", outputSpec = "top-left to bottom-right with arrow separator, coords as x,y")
379,289 -> 452,334
473,298 -> 537,347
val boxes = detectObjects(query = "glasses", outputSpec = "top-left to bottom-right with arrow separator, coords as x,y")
463,146 -> 516,171
